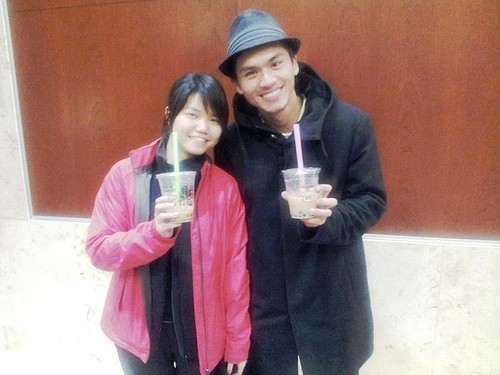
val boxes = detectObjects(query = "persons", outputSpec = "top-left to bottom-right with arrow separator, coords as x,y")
213,20 -> 388,375
85,71 -> 252,375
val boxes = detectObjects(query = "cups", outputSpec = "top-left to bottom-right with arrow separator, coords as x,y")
281,167 -> 322,220
156,170 -> 197,223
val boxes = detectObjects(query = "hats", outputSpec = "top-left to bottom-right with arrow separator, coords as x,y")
218,9 -> 301,78
